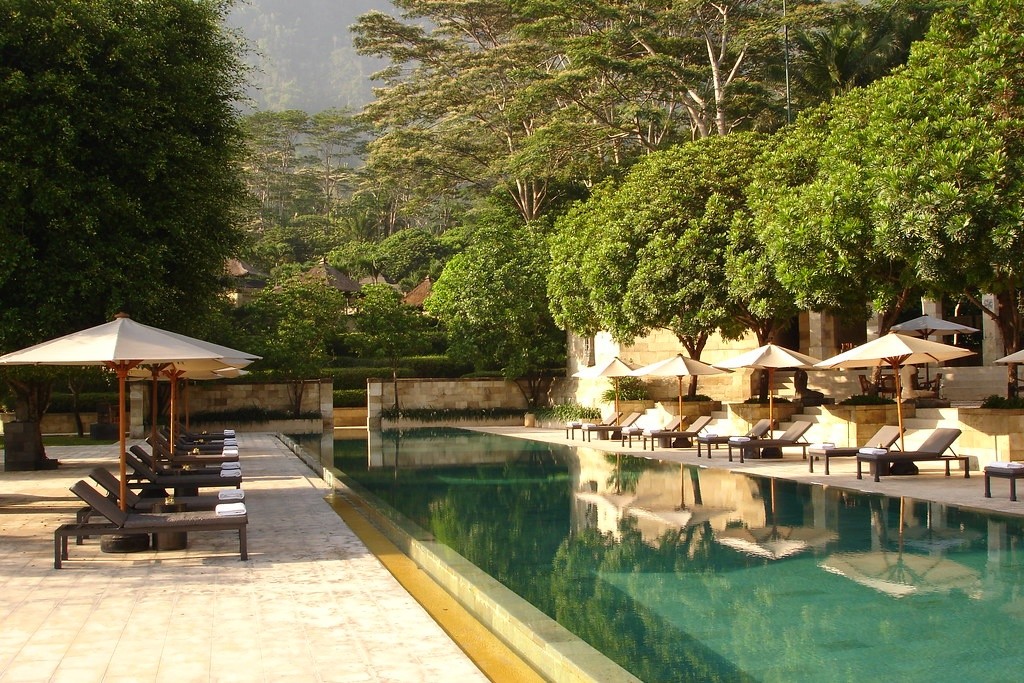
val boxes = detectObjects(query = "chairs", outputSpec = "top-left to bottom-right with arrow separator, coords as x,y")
54,421 -> 248,570
565,366 -> 1023,501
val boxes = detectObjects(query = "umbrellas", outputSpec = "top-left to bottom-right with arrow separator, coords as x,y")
813,332 -> 977,452
626,353 -> 736,432
571,357 -> 645,426
627,462 -> 738,529
993,350 -> 1024,365
0,312 -> 263,512
708,342 -> 824,439
817,497 -> 980,600
715,478 -> 839,560
890,314 -> 980,389
575,453 -> 662,512
